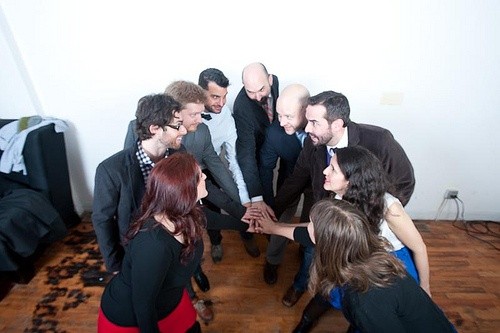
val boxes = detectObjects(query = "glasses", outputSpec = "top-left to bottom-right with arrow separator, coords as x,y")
164,120 -> 183,130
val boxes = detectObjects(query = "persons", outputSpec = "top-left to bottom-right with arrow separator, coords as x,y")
92,93 -> 262,320
123,81 -> 242,293
198,67 -> 253,264
97,152 -> 256,333
254,199 -> 458,333
270,145 -> 430,333
260,83 -> 314,285
234,61 -> 280,259
260,90 -> 416,307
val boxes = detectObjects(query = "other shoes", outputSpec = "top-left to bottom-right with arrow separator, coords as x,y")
264,263 -> 278,284
282,286 -> 303,307
193,297 -> 214,320
243,236 -> 260,257
292,316 -> 313,333
211,242 -> 223,262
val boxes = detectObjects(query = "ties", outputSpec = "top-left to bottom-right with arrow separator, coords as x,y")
264,97 -> 272,123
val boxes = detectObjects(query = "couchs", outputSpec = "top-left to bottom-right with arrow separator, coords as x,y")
0,118 -> 78,300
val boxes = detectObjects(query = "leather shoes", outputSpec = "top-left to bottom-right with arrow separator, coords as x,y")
194,272 -> 210,292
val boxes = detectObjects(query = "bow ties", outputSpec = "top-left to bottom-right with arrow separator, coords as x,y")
201,113 -> 212,121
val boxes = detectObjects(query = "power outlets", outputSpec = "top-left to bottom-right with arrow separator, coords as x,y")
445,190 -> 458,200
